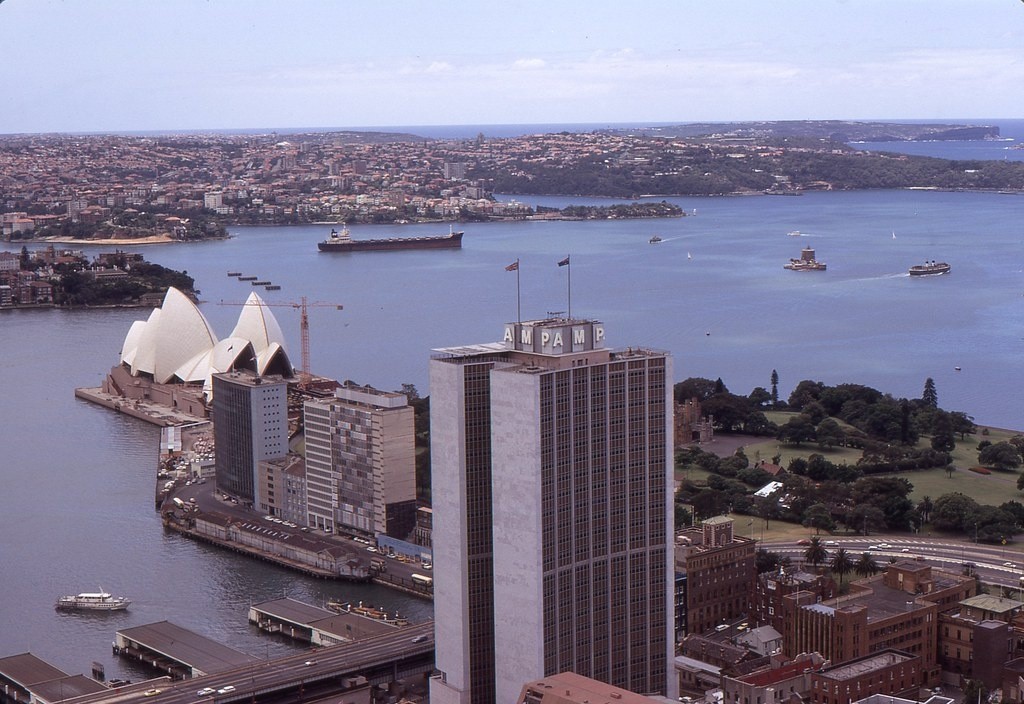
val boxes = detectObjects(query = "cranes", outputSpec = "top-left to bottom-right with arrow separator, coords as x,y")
214,292 -> 344,394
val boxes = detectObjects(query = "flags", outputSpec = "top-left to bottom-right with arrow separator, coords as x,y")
558,258 -> 569,267
504,262 -> 518,272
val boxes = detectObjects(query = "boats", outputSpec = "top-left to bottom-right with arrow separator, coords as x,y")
908,258 -> 952,276
317,219 -> 466,253
782,245 -> 827,272
649,236 -> 662,244
54,585 -> 134,613
787,230 -> 800,236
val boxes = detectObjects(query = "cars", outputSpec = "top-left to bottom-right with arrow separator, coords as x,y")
796,537 -> 1024,587
144,634 -> 431,698
